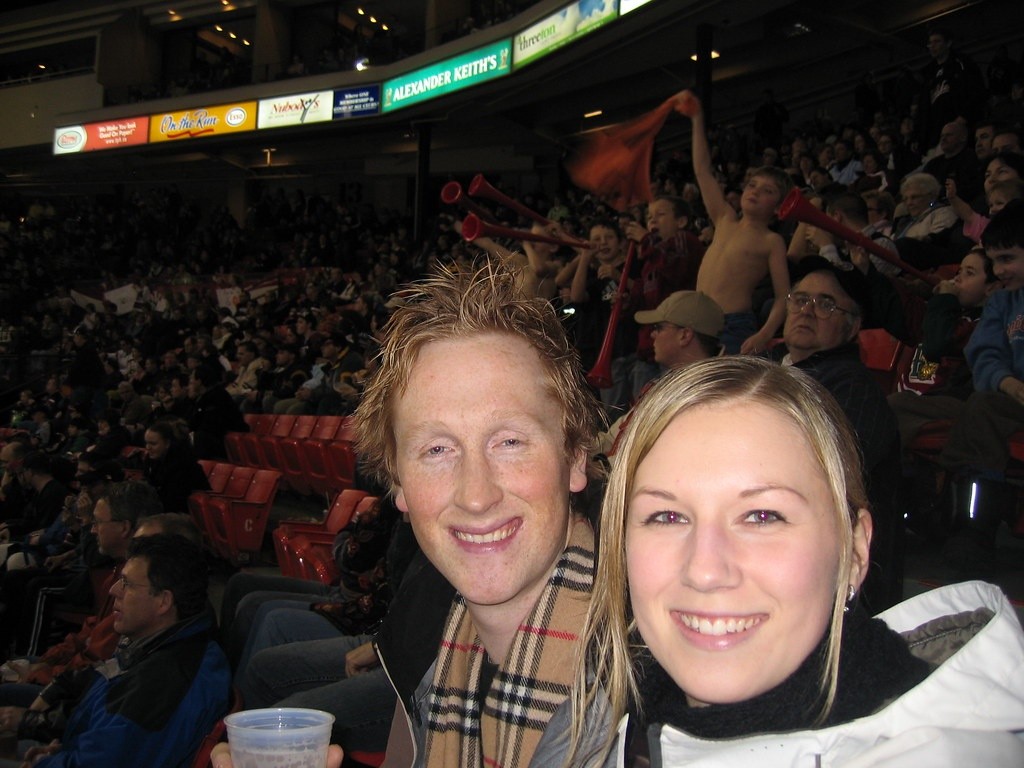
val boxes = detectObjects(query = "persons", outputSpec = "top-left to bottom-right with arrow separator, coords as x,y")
525,357 -> 1024,768
210,257 -> 640,768
0,17 -> 1024,768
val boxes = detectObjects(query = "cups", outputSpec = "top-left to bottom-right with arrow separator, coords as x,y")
223,708 -> 336,768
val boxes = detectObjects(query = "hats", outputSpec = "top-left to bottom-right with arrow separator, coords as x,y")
634,291 -> 724,339
797,255 -> 867,303
548,205 -> 569,221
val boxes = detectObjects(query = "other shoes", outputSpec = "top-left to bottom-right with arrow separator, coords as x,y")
939,535 -> 996,567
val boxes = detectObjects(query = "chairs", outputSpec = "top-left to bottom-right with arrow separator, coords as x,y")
122,326 -> 1024,768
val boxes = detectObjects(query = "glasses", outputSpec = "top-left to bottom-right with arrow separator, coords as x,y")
91,517 -> 115,528
785,294 -> 853,319
119,573 -> 151,590
653,325 -> 678,331
